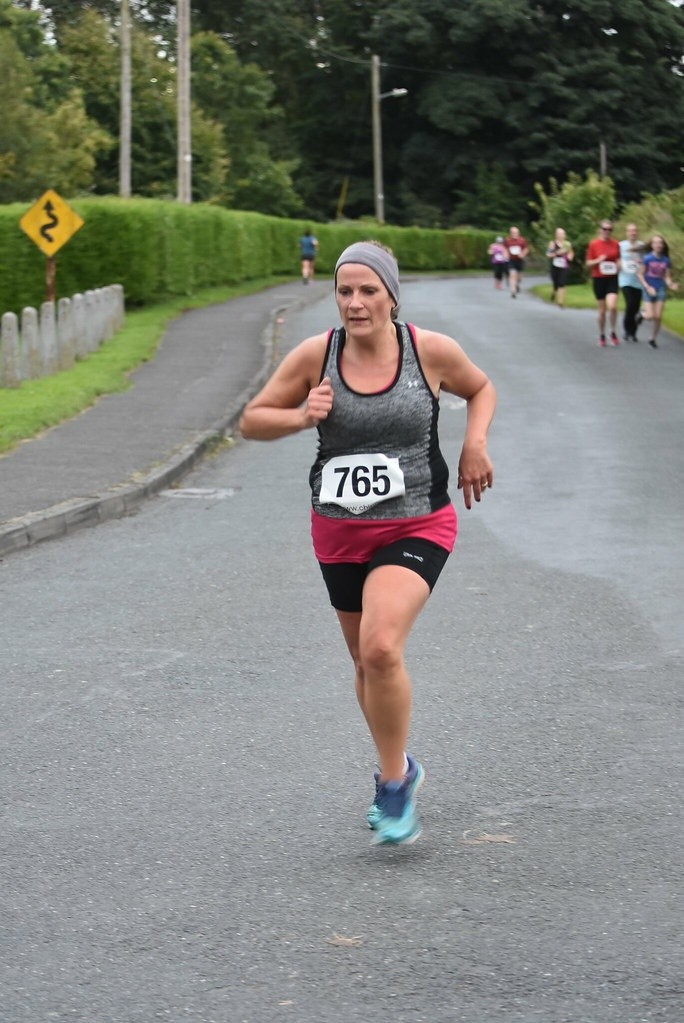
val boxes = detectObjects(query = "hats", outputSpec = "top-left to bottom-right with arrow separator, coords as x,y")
496,236 -> 504,243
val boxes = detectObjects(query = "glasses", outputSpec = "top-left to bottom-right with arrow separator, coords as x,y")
601,227 -> 613,232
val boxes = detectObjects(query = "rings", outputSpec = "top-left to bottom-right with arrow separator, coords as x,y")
482,481 -> 488,487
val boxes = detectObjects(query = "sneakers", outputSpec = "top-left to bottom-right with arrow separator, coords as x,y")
367,754 -> 426,846
609,332 -> 619,346
599,334 -> 605,347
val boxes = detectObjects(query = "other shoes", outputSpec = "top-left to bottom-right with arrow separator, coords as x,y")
630,334 -> 637,343
648,339 -> 658,349
635,310 -> 642,323
624,332 -> 630,341
303,277 -> 308,285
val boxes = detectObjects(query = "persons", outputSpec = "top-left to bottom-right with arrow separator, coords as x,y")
546,227 -> 574,307
618,224 -> 647,342
627,236 -> 678,351
585,220 -> 621,347
486,237 -> 510,290
239,240 -> 498,847
503,226 -> 530,297
297,228 -> 318,285
